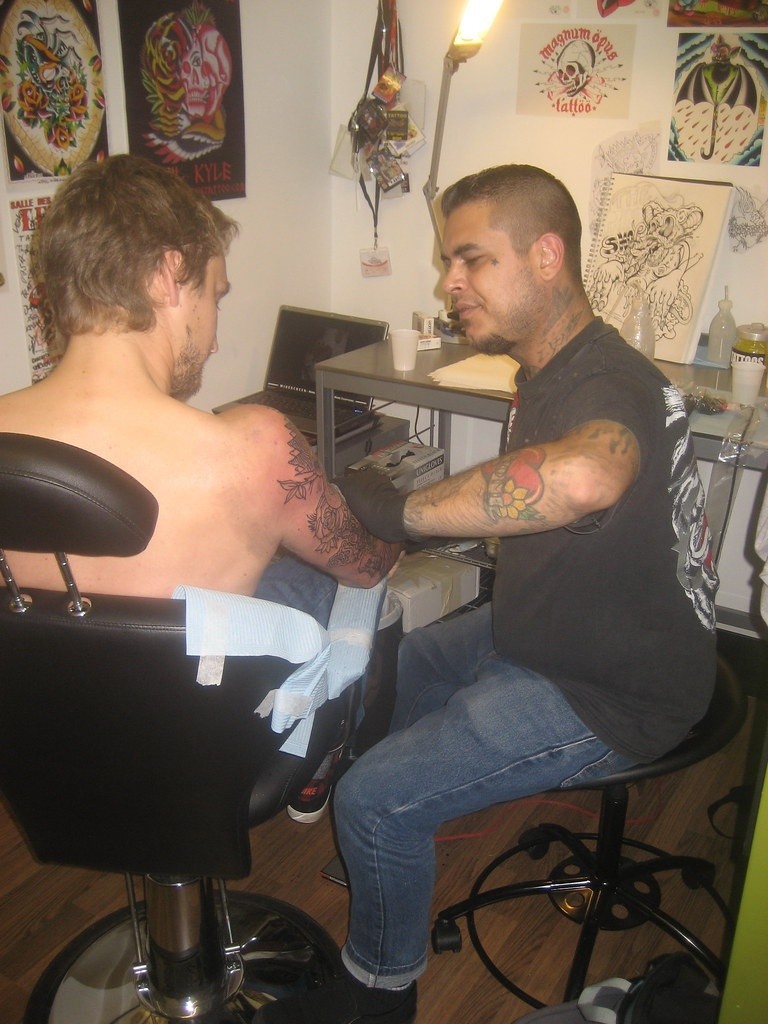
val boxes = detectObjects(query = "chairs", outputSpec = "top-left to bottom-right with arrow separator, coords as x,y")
0,430 -> 390,1024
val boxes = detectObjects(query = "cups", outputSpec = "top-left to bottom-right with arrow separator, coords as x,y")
390,329 -> 420,371
730,361 -> 766,409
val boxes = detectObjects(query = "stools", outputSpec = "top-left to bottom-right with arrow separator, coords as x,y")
431,646 -> 748,1010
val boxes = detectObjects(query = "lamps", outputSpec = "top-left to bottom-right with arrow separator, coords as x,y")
412,0 -> 505,344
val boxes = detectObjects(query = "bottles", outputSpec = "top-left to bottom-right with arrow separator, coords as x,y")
707,285 -> 736,364
618,298 -> 655,364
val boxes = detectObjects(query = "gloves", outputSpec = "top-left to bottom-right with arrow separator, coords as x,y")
332,467 -> 415,544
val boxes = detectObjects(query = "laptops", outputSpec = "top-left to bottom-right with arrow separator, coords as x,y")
212,304 -> 390,446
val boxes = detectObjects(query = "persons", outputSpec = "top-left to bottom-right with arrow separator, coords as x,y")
258,162 -> 722,1024
2,157 -> 408,831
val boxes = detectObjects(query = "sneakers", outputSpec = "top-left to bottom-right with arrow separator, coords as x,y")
287,748 -> 345,824
251,975 -> 418,1023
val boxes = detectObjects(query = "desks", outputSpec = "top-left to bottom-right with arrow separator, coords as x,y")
311,411 -> 409,478
312,330 -> 768,577
